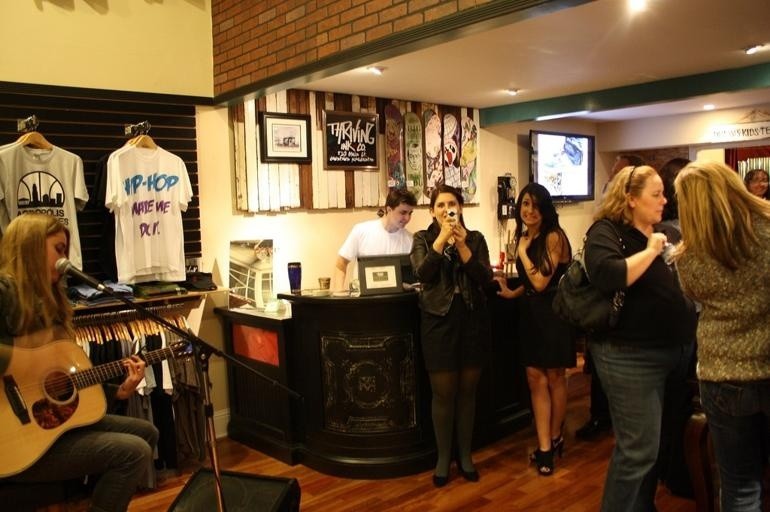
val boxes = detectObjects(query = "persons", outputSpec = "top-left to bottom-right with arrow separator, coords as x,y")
744,168 -> 770,202
583,163 -> 675,511
330,187 -> 416,290
492,181 -> 573,474
659,156 -> 700,502
664,159 -> 770,511
575,153 -> 647,439
233,243 -> 272,309
408,183 -> 495,488
0,212 -> 160,512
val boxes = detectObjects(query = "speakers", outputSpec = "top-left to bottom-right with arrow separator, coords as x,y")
167,466 -> 301,511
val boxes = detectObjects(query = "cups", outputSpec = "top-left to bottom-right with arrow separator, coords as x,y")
318,277 -> 330,289
287,261 -> 302,294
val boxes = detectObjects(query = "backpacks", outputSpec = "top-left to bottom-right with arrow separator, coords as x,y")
550,218 -> 625,344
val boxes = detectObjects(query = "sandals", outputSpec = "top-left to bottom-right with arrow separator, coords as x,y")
535,442 -> 552,477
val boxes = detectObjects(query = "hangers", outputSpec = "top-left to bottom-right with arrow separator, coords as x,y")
115,118 -> 167,156
10,113 -> 58,156
65,294 -> 193,354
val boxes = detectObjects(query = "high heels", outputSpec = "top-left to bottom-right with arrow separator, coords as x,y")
529,432 -> 564,462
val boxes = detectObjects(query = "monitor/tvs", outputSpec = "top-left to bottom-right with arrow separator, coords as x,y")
359,254 -> 417,285
528,128 -> 596,203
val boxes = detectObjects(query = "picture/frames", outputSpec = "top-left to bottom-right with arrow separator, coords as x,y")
321,108 -> 380,173
356,254 -> 404,296
257,110 -> 313,166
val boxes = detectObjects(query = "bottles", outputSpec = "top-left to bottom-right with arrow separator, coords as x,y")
661,237 -> 679,274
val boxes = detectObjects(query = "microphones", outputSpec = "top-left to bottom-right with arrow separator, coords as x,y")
54,258 -> 111,297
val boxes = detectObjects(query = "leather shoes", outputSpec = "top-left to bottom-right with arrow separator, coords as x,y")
455,455 -> 480,482
432,459 -> 451,488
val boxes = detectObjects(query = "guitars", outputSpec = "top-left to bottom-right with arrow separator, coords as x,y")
1,340 -> 189,477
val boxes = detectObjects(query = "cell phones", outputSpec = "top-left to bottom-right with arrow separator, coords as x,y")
447,209 -> 459,222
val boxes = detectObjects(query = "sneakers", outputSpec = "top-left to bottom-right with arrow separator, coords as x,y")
575,415 -> 608,441
